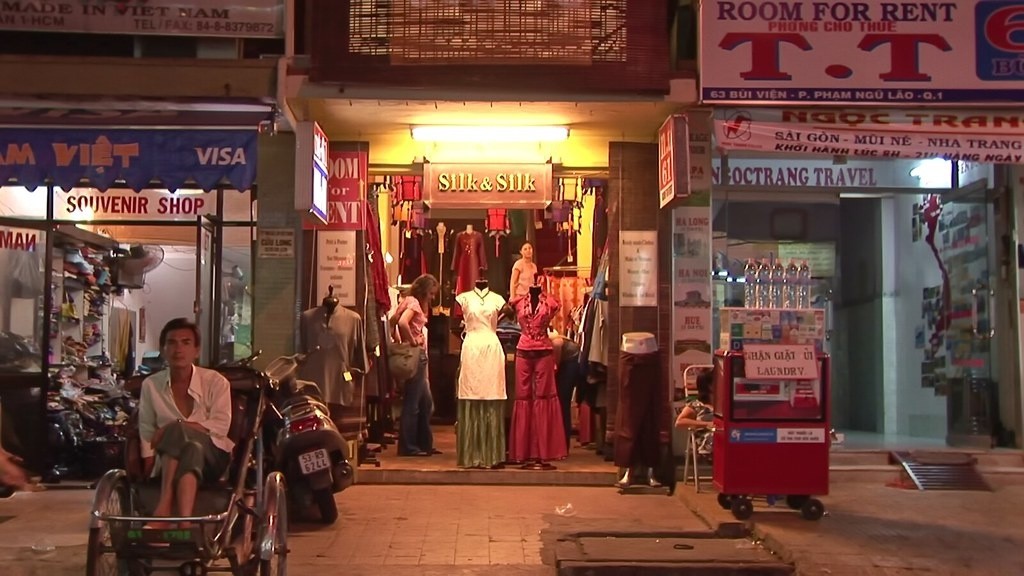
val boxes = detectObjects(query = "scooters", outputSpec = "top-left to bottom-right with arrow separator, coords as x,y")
209,345 -> 355,525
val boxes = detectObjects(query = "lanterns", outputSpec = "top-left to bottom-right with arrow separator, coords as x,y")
483,206 -> 510,256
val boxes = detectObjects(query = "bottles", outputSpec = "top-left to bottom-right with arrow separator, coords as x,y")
796,259 -> 812,309
757,258 -> 770,307
770,259 -> 783,308
744,258 -> 757,307
782,258 -> 798,308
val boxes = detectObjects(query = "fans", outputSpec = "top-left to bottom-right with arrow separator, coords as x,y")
111,241 -> 166,275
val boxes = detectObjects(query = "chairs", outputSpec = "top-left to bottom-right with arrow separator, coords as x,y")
681,364 -> 718,491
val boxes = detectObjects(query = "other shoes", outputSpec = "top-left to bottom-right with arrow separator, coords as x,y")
401,447 -> 442,457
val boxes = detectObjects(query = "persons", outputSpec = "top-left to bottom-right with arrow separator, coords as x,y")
676,369 -> 717,461
507,286 -> 568,470
137,317 -> 236,547
508,241 -> 537,302
299,294 -> 369,409
388,274 -> 445,457
617,331 -> 670,487
452,279 -> 510,467
427,223 -> 489,316
549,332 -> 583,450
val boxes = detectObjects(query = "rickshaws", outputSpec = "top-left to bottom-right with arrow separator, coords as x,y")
85,365 -> 288,576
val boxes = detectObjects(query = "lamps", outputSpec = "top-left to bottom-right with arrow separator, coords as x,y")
220,263 -> 246,282
408,124 -> 570,142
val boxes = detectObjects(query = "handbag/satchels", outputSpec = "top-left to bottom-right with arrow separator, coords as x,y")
387,342 -> 421,379
